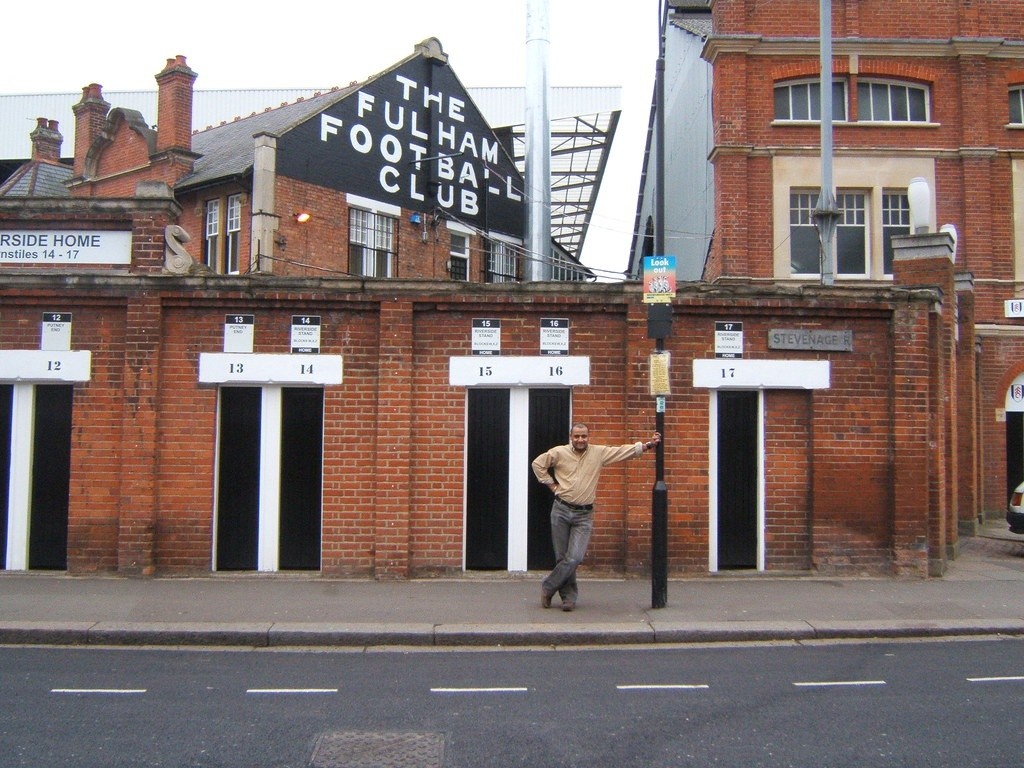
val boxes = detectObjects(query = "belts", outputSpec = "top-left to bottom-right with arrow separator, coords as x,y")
556,495 -> 593,510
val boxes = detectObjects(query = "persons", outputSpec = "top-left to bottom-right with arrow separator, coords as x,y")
531,423 -> 661,612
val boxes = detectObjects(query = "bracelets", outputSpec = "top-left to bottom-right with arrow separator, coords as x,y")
646,442 -> 652,449
552,485 -> 558,492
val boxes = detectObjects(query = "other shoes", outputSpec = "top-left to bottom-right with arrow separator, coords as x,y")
541,577 -> 551,607
563,603 -> 575,610
